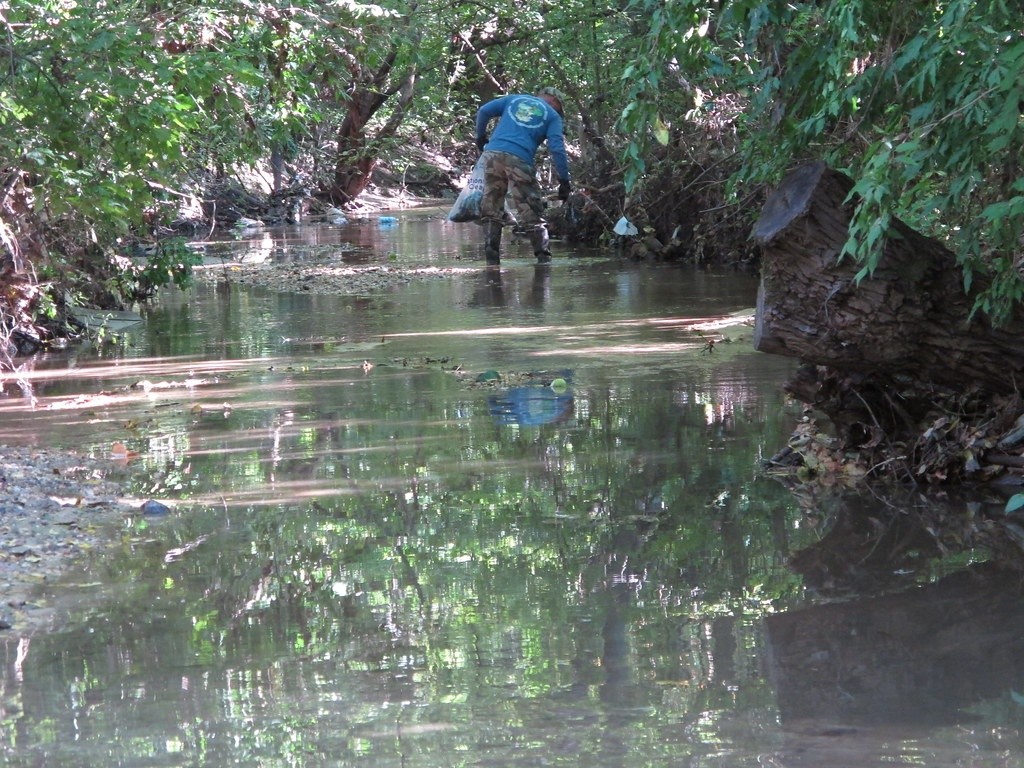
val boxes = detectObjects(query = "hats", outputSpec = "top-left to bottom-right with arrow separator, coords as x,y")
537,91 -> 564,116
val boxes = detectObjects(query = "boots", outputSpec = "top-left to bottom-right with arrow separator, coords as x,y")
524,219 -> 551,264
484,221 -> 501,265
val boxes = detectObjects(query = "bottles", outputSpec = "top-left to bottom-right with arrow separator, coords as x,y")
378,217 -> 399,223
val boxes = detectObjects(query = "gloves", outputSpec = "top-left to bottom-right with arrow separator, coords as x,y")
559,179 -> 571,204
475,136 -> 488,152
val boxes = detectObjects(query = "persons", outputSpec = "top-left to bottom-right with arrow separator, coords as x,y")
466,266 -> 574,458
475,86 -> 571,264
